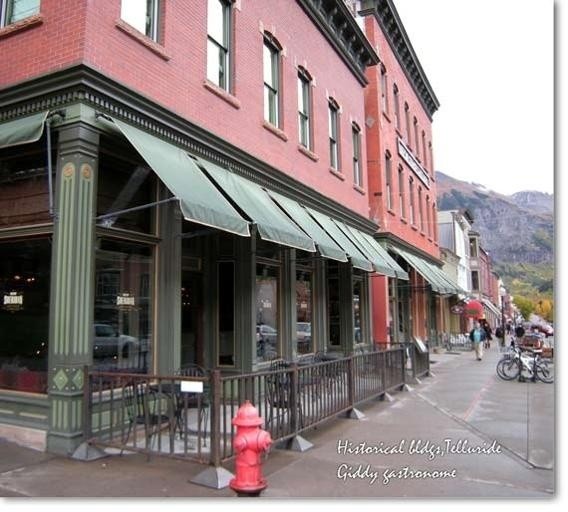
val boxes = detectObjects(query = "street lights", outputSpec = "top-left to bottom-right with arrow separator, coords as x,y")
499,287 -> 506,353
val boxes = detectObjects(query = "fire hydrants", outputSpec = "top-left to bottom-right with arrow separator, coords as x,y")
230,399 -> 274,497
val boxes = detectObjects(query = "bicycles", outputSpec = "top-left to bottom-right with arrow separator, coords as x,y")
496,336 -> 554,383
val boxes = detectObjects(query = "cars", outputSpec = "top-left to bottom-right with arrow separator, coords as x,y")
93,324 -> 138,360
255,323 -> 278,347
297,321 -> 312,346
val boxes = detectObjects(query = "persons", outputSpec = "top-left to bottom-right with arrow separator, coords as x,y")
468,319 -> 544,362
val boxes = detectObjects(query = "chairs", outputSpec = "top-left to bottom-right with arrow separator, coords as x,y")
123,352 -> 343,455
